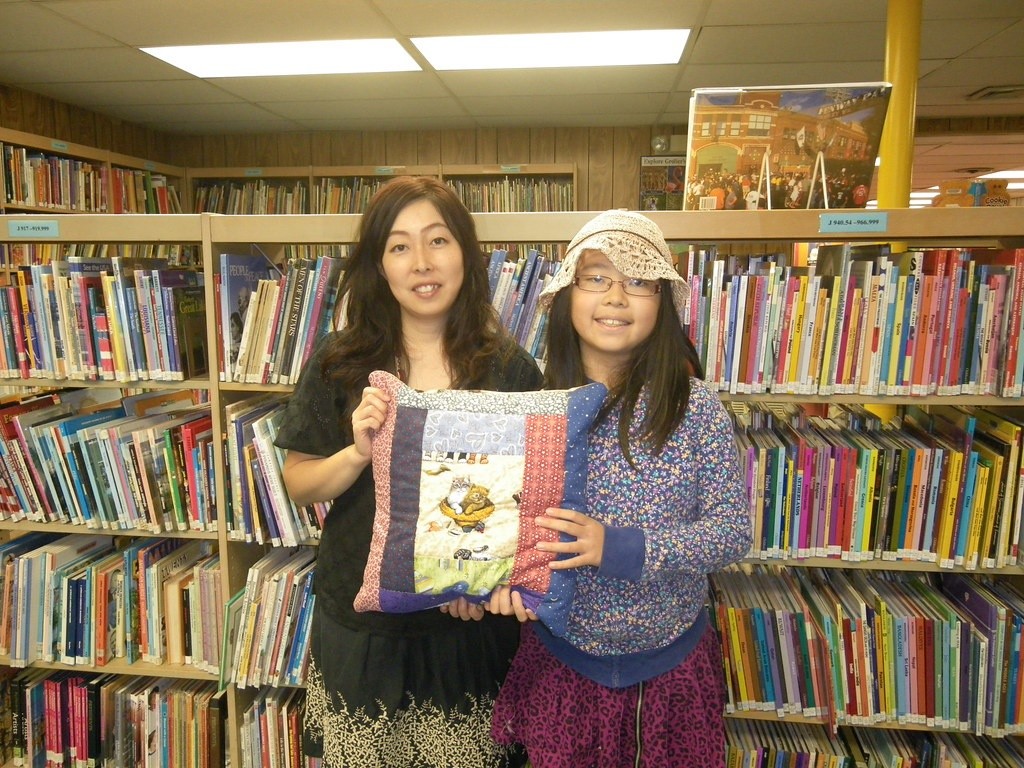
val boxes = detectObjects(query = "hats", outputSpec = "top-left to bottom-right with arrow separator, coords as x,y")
537,209 -> 689,319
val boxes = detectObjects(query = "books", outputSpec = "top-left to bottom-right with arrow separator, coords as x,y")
0,241 -> 1024,768
2,142 -> 575,214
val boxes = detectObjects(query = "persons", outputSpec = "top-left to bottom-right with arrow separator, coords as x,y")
273,177 -> 543,768
485,211 -> 753,768
684,167 -> 871,210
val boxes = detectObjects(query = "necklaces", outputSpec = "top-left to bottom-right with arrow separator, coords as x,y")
393,341 -> 401,379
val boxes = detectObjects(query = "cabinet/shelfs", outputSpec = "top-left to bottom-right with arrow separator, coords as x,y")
185,161 -> 577,214
0,206 -> 1024,768
0,127 -> 187,214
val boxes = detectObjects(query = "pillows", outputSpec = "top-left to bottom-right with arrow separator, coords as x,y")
355,372 -> 608,637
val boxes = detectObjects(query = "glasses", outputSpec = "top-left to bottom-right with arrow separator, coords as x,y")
571,274 -> 666,296
